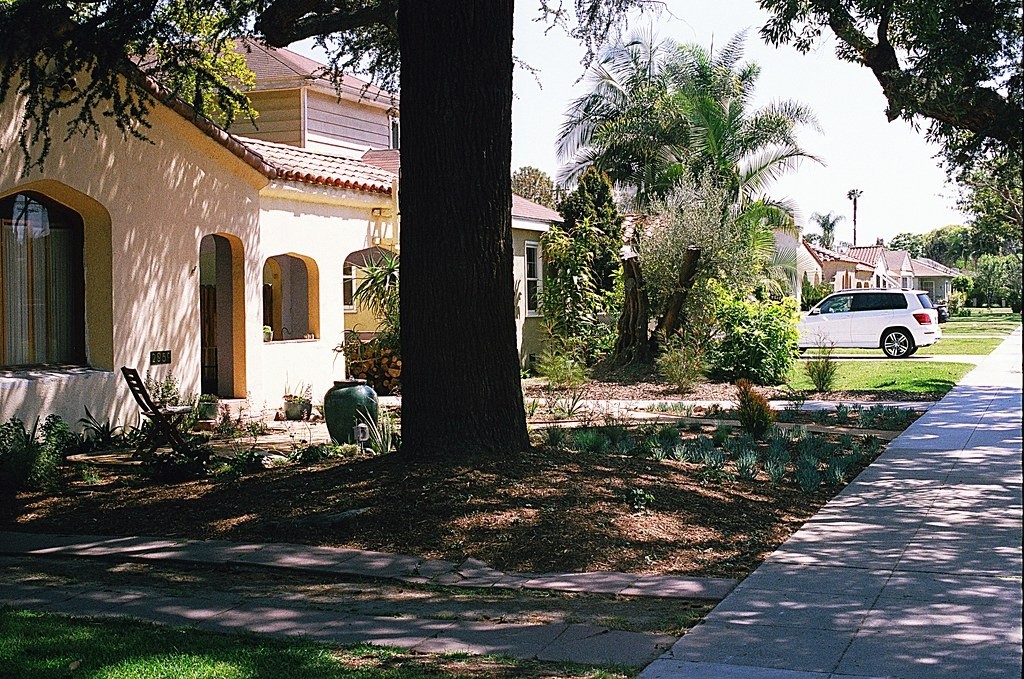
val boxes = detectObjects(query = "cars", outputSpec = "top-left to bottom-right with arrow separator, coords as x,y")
933,303 -> 950,323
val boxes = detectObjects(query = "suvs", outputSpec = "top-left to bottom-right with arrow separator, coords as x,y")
788,288 -> 943,359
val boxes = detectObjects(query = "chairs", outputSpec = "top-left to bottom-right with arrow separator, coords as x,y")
119,366 -> 196,461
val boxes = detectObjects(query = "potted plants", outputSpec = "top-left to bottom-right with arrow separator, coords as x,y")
281,371 -> 314,421
197,393 -> 220,419
263,324 -> 274,342
